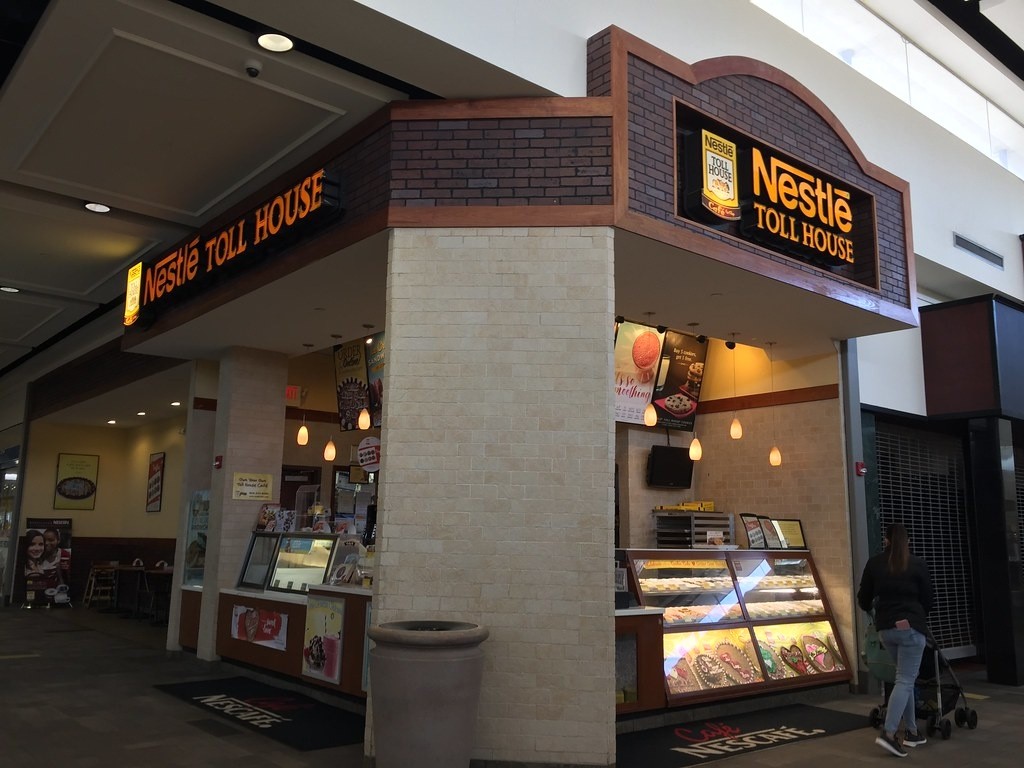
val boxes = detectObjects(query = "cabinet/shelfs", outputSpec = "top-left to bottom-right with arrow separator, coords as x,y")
655,507 -> 735,580
603,548 -> 854,704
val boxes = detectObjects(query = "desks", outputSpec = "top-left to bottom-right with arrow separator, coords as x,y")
97,565 -> 144,622
148,567 -> 172,628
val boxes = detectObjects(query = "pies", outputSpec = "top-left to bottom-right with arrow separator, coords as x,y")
57,477 -> 97,498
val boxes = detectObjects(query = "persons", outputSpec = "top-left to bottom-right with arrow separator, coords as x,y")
857,524 -> 933,757
24,529 -> 71,577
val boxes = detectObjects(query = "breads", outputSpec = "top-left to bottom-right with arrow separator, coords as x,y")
638,577 -> 823,622
707,537 -> 723,545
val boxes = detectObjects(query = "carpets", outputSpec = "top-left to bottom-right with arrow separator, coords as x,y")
155,676 -> 365,752
616,703 -> 881,768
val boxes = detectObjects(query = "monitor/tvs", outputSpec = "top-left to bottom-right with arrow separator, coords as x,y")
647,445 -> 694,489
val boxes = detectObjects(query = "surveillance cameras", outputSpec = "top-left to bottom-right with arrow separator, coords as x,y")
244,59 -> 263,77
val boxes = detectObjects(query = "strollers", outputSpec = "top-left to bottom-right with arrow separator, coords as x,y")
861,601 -> 979,741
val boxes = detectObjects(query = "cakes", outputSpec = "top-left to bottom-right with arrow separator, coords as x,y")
664,632 -> 845,696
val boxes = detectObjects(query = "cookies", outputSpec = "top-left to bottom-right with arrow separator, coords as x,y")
685,362 -> 704,397
664,394 -> 692,414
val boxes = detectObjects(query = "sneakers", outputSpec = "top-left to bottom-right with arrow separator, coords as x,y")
903,729 -> 926,747
875,732 -> 908,757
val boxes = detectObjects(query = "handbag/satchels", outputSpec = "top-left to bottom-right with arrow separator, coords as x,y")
860,613 -> 897,682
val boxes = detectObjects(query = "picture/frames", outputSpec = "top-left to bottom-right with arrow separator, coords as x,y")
54,452 -> 100,511
146,451 -> 165,513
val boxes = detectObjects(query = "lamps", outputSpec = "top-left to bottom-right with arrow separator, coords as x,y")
323,333 -> 343,462
762,339 -> 783,466
687,321 -> 704,461
642,311 -> 659,426
359,324 -> 376,430
295,401 -> 309,448
729,331 -> 743,440
